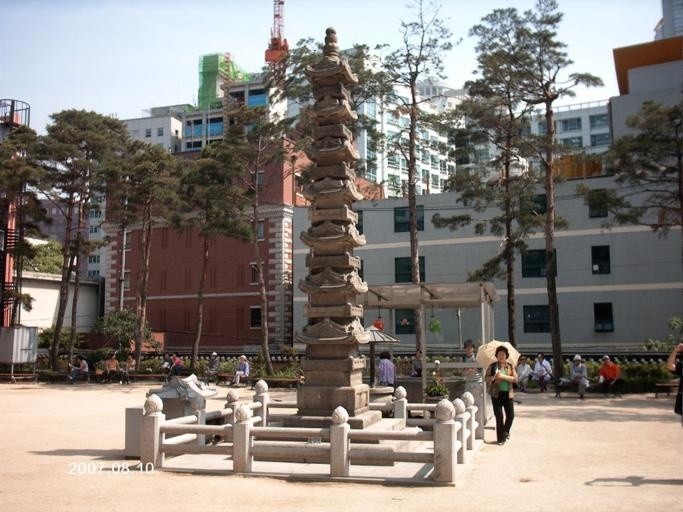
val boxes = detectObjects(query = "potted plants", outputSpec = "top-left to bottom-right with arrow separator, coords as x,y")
424,377 -> 448,403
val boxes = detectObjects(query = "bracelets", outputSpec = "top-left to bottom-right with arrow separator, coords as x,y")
672,349 -> 678,353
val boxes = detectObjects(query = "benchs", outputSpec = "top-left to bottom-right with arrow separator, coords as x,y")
501,374 -> 626,398
2,369 -> 297,385
654,381 -> 683,399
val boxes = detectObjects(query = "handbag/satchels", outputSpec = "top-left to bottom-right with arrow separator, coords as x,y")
513,383 -> 521,389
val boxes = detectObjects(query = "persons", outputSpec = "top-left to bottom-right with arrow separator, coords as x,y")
411,349 -> 422,376
667,343 -> 683,427
571,354 -> 590,399
516,353 -> 534,393
456,339 -> 483,376
68,352 -> 249,385
484,346 -> 519,444
533,353 -> 552,392
376,351 -> 397,385
598,355 -> 622,398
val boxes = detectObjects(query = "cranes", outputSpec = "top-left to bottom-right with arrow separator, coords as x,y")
262,0 -> 290,79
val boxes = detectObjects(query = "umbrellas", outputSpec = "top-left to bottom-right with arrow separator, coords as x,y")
475,339 -> 522,370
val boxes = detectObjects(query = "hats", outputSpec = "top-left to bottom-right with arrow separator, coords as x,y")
573,355 -> 585,361
240,355 -> 247,361
212,352 -> 218,356
602,356 -> 609,360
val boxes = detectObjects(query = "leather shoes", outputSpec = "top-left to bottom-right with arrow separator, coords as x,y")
504,429 -> 509,439
498,443 -> 504,445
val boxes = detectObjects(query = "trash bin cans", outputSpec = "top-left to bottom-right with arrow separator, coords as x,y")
126,408 -> 145,459
